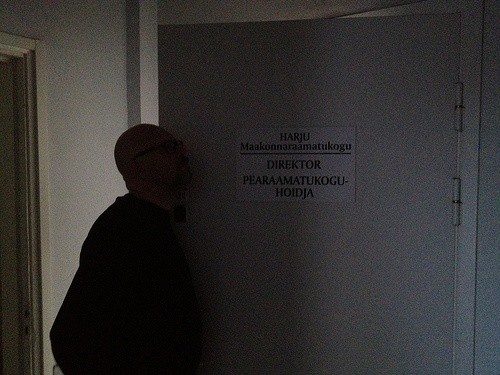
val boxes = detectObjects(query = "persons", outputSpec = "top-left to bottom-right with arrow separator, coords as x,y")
49,123 -> 204,375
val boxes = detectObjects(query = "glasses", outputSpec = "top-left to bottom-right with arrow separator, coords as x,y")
129,143 -> 184,160
